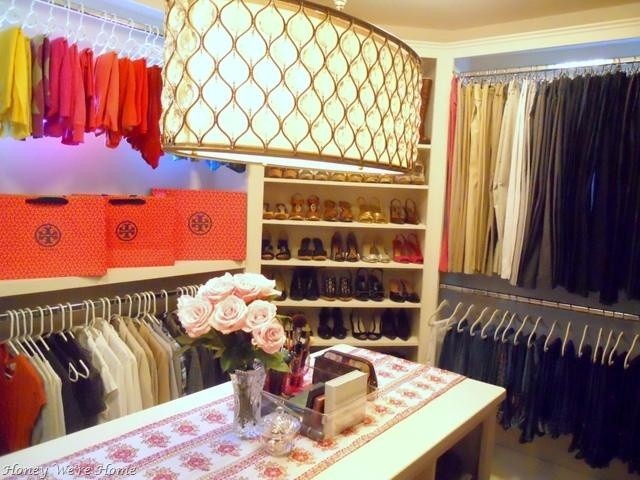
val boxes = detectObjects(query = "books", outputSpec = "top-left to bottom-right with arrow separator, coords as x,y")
284,368 -> 370,440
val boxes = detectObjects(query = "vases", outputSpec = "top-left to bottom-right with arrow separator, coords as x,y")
223,359 -> 270,440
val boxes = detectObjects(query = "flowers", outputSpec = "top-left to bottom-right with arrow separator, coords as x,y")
175,270 -> 292,371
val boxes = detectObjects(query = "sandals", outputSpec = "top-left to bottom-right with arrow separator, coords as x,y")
263,160 -> 426,340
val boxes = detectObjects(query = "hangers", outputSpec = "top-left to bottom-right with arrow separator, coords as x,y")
454,58 -> 640,94
0,283 -> 219,393
426,282 -> 640,371
0,0 -> 166,73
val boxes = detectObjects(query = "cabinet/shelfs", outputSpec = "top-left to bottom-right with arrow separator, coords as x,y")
0,0 -> 250,450
247,37 -> 451,376
436,20 -> 639,480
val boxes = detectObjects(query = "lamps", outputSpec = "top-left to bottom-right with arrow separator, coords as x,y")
160,1 -> 423,177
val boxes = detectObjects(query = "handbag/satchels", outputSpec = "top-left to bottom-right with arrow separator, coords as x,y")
151,187 -> 246,260
313,349 -> 380,393
100,194 -> 177,267
0,192 -> 106,278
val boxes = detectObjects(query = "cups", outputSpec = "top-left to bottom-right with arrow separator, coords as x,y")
254,407 -> 300,455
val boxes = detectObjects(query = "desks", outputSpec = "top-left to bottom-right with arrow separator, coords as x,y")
0,346 -> 506,480
